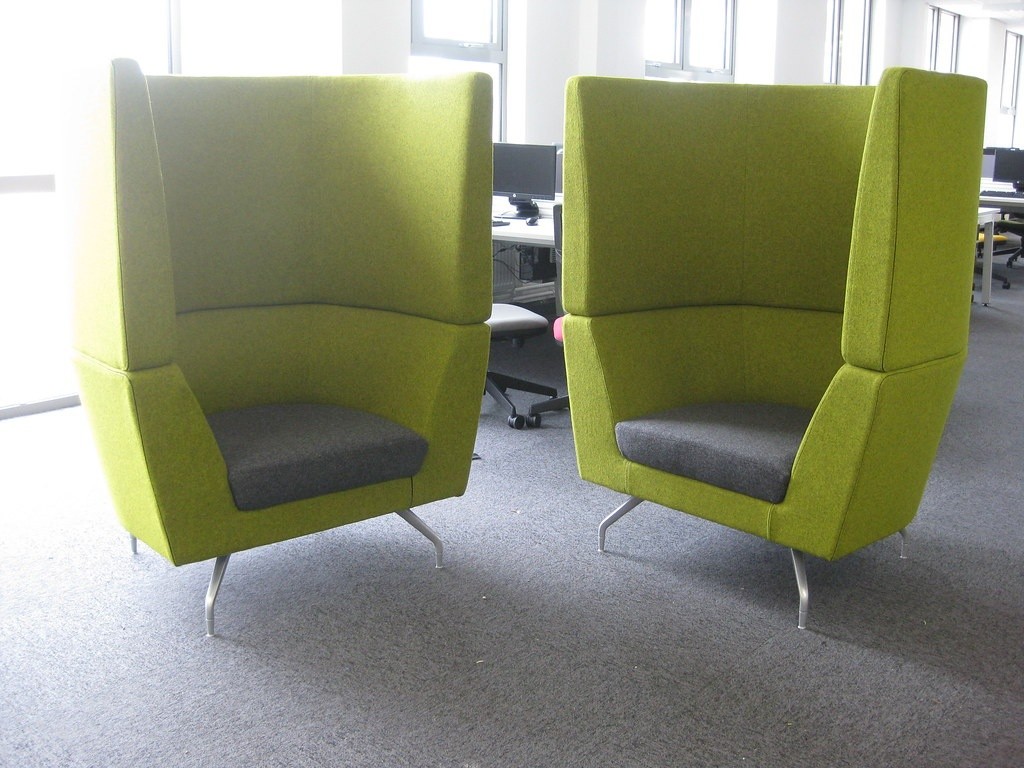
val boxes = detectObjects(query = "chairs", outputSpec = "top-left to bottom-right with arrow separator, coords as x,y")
526,312 -> 573,429
482,299 -> 559,428
967,203 -> 1024,310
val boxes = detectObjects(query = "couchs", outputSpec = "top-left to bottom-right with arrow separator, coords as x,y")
562,67 -> 989,629
72,57 -> 493,638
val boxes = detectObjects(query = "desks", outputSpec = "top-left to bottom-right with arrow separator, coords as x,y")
493,190 -> 565,247
979,178 -> 1024,209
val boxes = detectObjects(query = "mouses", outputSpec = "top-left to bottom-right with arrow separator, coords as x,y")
526,217 -> 538,226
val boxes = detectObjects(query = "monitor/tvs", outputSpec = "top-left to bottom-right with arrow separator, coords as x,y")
993,149 -> 1024,193
492,143 -> 557,219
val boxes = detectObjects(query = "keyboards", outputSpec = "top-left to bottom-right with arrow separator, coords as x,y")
493,220 -> 510,226
980,191 -> 1024,198
